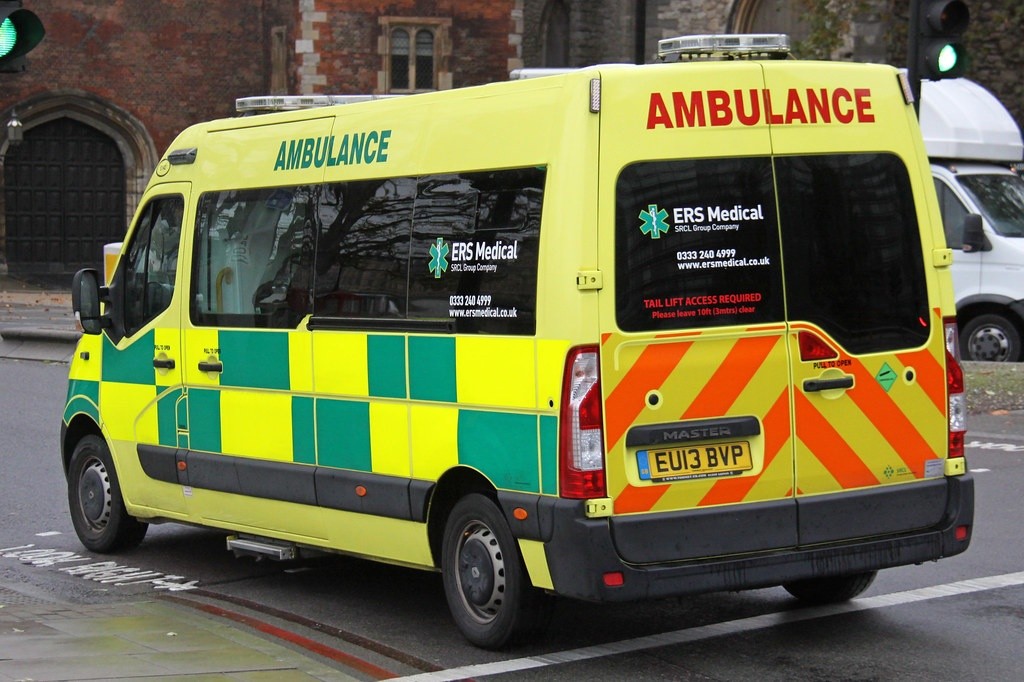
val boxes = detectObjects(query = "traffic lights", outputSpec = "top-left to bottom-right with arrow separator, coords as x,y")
0,0 -> 48,69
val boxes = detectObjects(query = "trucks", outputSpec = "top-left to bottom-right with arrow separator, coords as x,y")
912,71 -> 1024,366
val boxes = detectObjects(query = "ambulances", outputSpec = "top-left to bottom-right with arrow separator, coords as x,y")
57,31 -> 977,654
